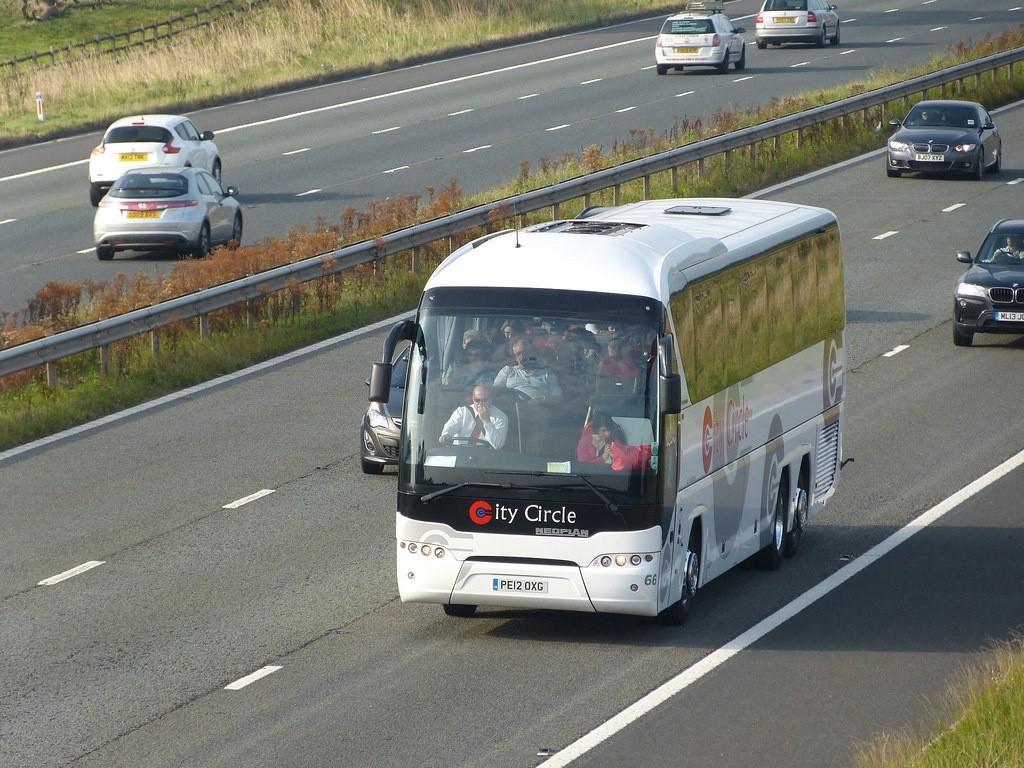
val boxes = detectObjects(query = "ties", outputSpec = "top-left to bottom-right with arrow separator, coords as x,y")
468,415 -> 483,446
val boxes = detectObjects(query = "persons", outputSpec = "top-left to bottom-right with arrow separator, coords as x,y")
577,412 -> 622,464
911,111 -> 930,125
439,385 -> 509,450
442,317 -> 644,456
992,236 -> 1024,263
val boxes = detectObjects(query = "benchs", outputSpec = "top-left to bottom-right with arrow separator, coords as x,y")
425,385 -> 531,401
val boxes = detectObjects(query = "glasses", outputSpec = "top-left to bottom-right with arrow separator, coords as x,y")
473,398 -> 490,403
513,351 -> 532,356
566,340 -> 577,342
466,352 -> 481,357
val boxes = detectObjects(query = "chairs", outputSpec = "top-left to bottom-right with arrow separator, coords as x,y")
774,0 -> 787,10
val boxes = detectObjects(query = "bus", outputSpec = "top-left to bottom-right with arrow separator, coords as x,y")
366,196 -> 855,626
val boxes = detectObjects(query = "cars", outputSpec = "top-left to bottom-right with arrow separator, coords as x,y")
886,99 -> 1001,181
655,1 -> 747,75
755,0 -> 841,50
360,345 -> 439,465
92,166 -> 244,261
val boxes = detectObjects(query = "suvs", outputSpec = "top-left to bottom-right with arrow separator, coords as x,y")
88,114 -> 222,207
952,218 -> 1024,347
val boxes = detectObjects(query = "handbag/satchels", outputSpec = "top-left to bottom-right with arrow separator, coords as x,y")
611,440 -> 653,471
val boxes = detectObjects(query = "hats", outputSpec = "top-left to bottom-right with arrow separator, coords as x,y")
463,331 -> 482,344
